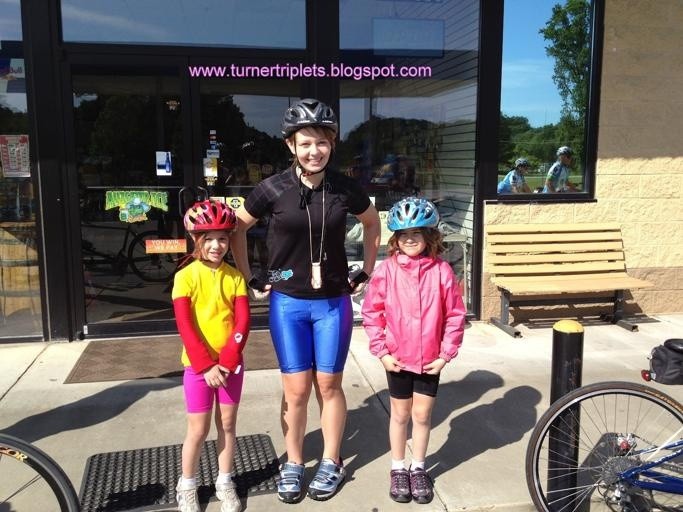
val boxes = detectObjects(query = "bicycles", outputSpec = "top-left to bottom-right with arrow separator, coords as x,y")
77,199 -> 184,283
0,434 -> 79,511
525,335 -> 683,512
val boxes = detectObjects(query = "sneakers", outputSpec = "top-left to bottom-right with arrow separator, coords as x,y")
176,478 -> 202,512
307,458 -> 347,501
277,462 -> 305,503
214,482 -> 243,512
390,468 -> 412,503
409,464 -> 434,504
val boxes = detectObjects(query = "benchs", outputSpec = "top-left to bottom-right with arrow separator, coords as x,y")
482,220 -> 655,339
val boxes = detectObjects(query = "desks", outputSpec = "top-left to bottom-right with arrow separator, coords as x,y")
441,231 -> 468,315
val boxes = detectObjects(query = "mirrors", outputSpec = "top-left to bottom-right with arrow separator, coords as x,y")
495,0 -> 605,202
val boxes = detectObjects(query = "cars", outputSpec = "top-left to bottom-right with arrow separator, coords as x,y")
370,162 -> 401,189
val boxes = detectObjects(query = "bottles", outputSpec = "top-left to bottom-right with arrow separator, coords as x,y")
164,153 -> 171,174
343,167 -> 353,176
209,129 -> 216,150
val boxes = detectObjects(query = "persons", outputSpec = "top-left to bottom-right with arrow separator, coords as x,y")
363,196 -> 467,503
167,198 -> 251,512
497,158 -> 535,193
232,98 -> 383,503
541,145 -> 581,195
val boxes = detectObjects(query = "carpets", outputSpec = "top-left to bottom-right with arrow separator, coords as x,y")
79,435 -> 281,512
63,329 -> 288,382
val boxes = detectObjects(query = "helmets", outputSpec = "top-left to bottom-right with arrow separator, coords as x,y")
184,199 -> 239,238
557,146 -> 574,156
514,159 -> 532,168
281,98 -> 338,138
388,198 -> 441,231
